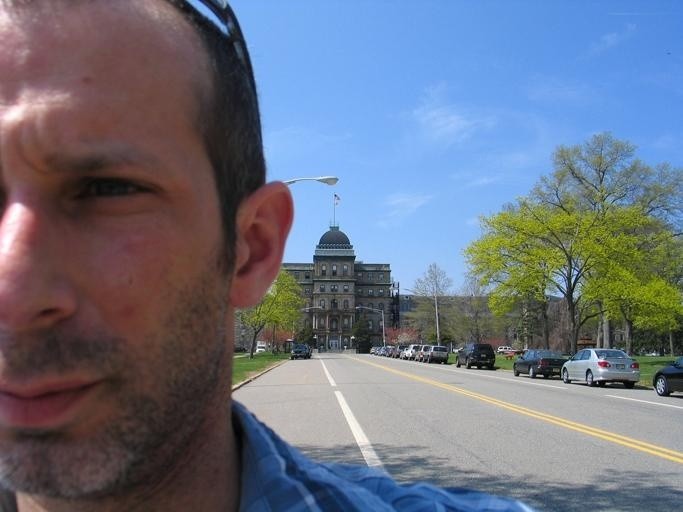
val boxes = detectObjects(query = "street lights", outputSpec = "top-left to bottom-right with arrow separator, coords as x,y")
355,306 -> 386,347
283,175 -> 338,188
387,284 -> 439,346
290,306 -> 323,357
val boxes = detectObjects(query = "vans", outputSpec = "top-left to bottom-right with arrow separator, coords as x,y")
496,346 -> 513,353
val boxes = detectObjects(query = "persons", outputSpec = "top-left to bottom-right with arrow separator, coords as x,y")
0,0 -> 532,512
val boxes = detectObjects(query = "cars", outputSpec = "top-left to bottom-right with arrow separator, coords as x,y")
644,352 -> 660,357
369,345 -> 449,363
452,347 -> 463,354
651,356 -> 683,396
560,347 -> 641,389
513,349 -> 569,379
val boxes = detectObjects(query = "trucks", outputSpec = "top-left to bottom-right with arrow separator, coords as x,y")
255,340 -> 264,353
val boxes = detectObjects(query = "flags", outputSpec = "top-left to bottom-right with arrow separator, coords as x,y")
334,193 -> 340,204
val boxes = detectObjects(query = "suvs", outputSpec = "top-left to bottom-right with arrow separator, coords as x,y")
456,343 -> 495,370
291,344 -> 311,359
